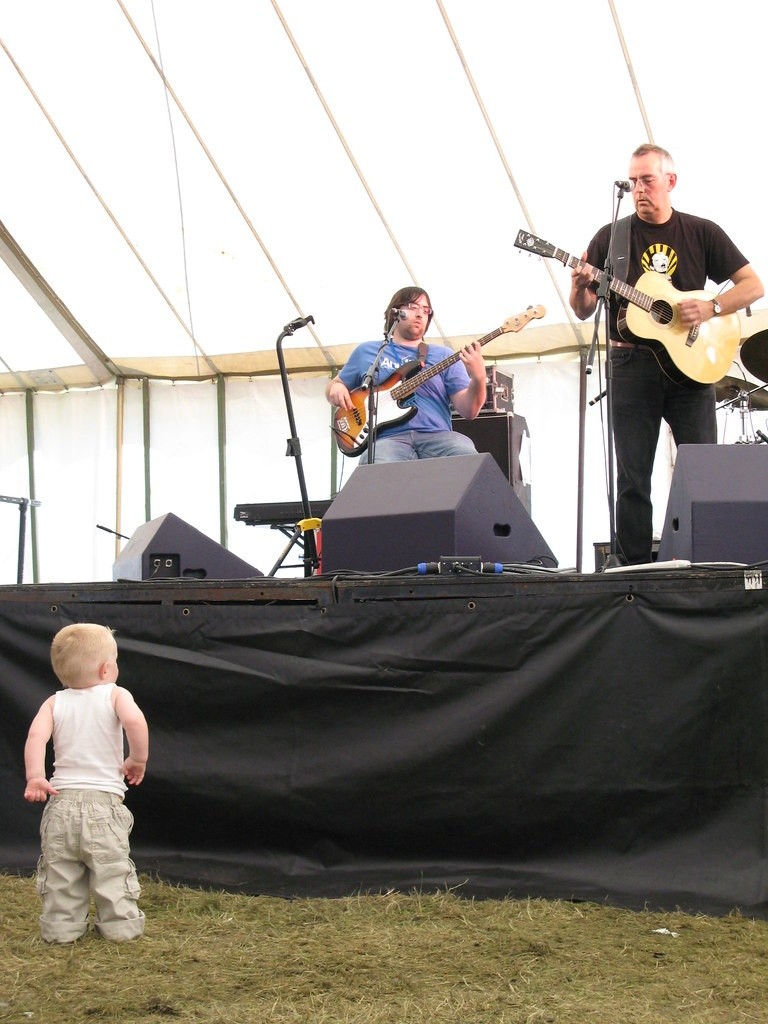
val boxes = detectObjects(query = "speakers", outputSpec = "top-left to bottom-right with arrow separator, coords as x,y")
112,513 -> 264,580
321,452 -> 559,569
657,442 -> 768,569
451,411 -> 532,519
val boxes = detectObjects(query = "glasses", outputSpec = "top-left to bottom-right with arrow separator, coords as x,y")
405,303 -> 432,314
634,176 -> 667,185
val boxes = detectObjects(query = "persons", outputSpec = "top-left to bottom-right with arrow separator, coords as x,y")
326,287 -> 488,465
23,623 -> 149,944
569,144 -> 765,567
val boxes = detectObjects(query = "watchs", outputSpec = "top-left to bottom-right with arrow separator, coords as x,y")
710,299 -> 721,317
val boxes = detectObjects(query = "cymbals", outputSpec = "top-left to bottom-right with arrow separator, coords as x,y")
715,375 -> 768,412
739,328 -> 768,385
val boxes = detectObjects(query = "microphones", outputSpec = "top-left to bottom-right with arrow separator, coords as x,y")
615,179 -> 635,192
391,308 -> 410,320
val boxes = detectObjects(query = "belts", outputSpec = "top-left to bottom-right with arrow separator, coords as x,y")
609,339 -> 650,349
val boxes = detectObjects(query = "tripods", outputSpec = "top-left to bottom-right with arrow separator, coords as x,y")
268,316 -> 323,577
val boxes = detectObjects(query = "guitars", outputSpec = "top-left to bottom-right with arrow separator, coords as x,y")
331,303 -> 547,458
513,228 -> 743,391
